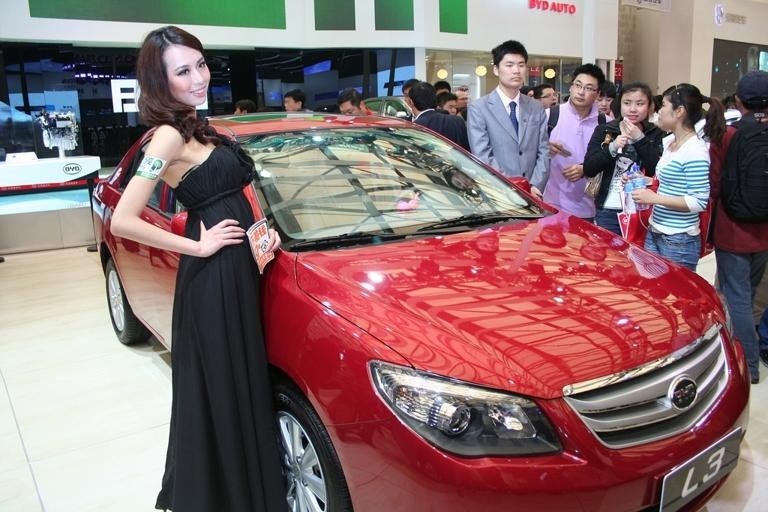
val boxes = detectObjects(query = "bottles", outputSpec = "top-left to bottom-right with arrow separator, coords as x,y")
630,165 -> 649,211
623,173 -> 636,214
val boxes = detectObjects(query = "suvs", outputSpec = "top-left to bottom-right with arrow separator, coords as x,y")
357,96 -> 418,121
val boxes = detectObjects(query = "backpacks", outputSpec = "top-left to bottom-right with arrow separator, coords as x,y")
616,126 -> 716,258
722,114 -> 768,223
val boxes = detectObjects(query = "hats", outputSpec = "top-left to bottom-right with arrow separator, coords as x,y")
736,68 -> 768,108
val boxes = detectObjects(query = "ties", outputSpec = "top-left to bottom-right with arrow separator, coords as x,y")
509,101 -> 518,137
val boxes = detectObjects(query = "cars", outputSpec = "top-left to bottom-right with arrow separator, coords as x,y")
92,112 -> 751,512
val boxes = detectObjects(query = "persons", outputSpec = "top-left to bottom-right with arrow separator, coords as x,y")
620,84 -> 727,272
110,27 -> 291,512
639,91 -> 743,145
283,89 -> 313,112
520,83 -> 560,110
468,41 -> 551,202
709,70 -> 768,385
407,82 -> 471,153
455,86 -> 469,119
336,87 -> 374,115
234,99 -> 255,114
583,81 -> 667,241
436,93 -> 458,115
402,78 -> 420,122
595,80 -> 615,119
543,64 -> 614,226
434,82 -> 451,96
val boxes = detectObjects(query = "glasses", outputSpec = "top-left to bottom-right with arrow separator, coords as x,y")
571,83 -> 599,92
